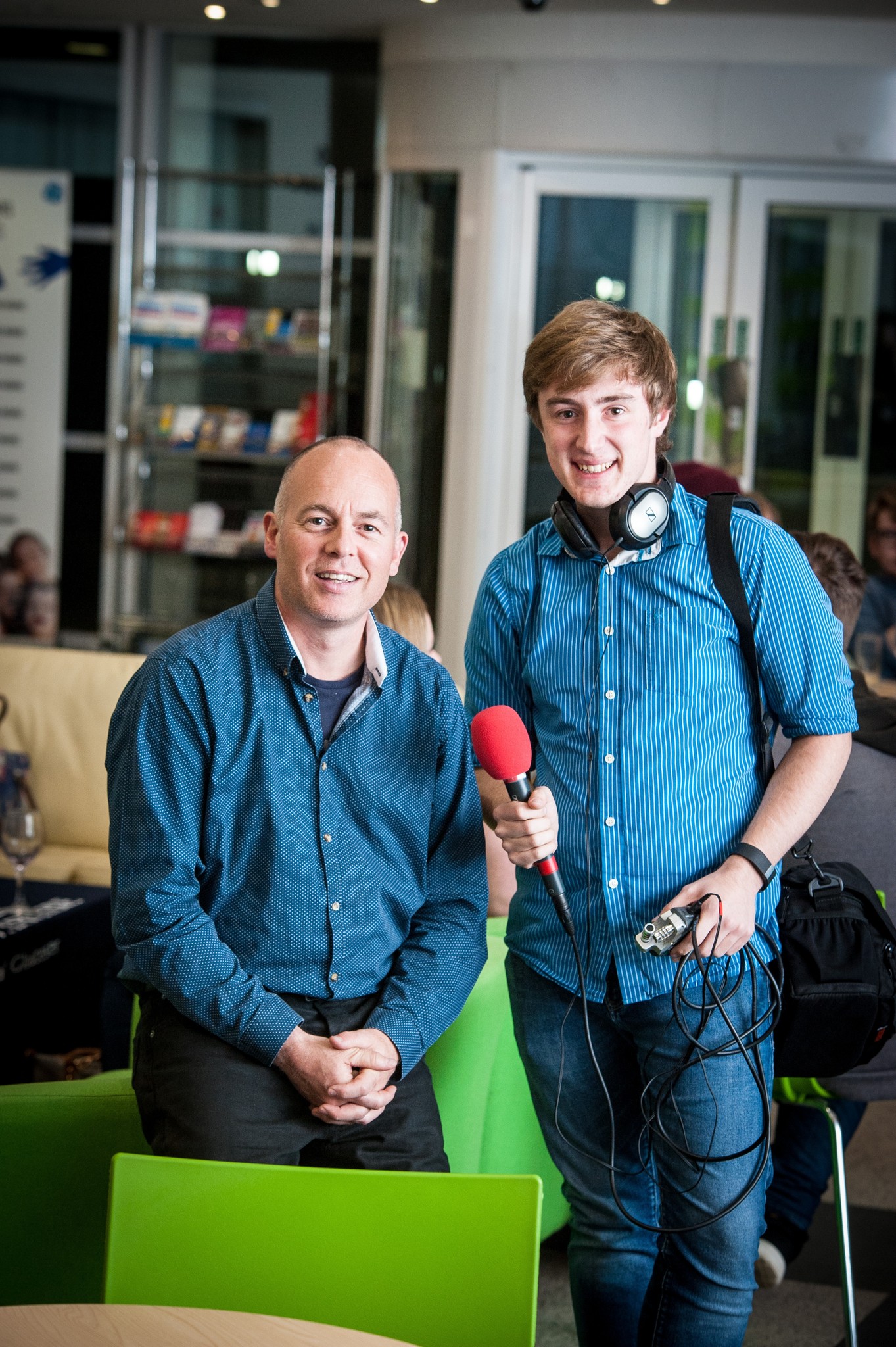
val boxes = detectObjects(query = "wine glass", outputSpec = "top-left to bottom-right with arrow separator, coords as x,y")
1,808 -> 46,917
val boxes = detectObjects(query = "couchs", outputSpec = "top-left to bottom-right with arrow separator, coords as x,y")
0,995 -> 158,1307
0,641 -> 155,890
421,911 -> 579,1253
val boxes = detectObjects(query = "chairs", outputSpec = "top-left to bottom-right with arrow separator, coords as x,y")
104,1150 -> 543,1347
772,884 -> 896,1347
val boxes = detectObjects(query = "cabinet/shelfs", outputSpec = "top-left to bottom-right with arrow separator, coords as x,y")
95,145 -> 339,669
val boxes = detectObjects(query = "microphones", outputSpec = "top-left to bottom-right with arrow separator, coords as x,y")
472,705 -> 574,937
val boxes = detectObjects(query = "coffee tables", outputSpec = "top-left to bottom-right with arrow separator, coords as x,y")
0,873 -> 132,1086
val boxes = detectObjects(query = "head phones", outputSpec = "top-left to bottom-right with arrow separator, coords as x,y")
550,455 -> 677,560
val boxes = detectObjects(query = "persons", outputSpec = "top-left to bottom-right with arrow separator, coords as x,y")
849,489 -> 896,680
104,435 -> 491,1177
1,532 -> 59,644
749,528 -> 896,1291
463,297 -> 860,1346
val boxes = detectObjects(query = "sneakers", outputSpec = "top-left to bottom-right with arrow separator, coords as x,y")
755,1219 -> 799,1286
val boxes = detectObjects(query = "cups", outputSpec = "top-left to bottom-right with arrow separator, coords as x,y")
852,632 -> 885,697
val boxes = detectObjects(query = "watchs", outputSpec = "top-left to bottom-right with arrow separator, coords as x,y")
727,842 -> 777,893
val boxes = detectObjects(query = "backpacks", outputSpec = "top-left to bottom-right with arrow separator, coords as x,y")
773,855 -> 896,1076
